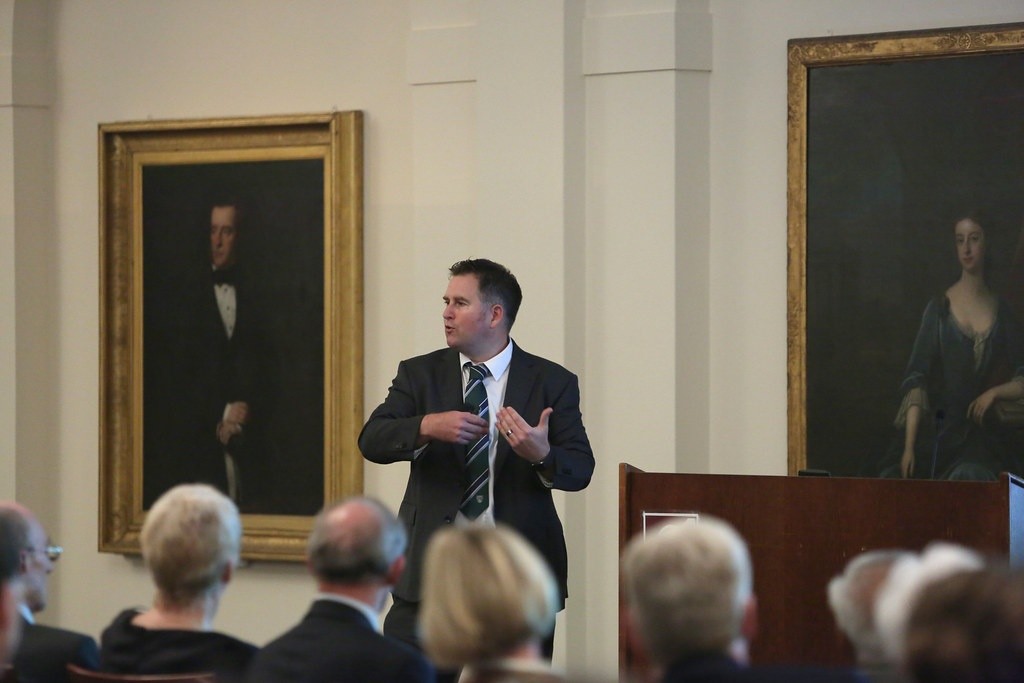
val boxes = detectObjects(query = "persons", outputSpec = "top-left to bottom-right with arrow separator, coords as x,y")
357,258 -> 595,683
417,520 -> 595,683
99,480 -> 261,683
243,494 -> 437,683
0,513 -> 33,679
0,500 -> 99,683
620,512 -> 867,683
826,541 -> 1024,683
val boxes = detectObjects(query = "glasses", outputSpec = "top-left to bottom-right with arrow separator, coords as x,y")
27,546 -> 60,561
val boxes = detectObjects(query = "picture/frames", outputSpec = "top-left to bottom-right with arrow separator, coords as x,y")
98,110 -> 363,566
788,22 -> 1024,481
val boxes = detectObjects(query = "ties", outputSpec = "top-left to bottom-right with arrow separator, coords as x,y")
459,362 -> 492,521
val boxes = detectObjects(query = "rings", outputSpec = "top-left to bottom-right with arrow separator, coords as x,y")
506,429 -> 513,437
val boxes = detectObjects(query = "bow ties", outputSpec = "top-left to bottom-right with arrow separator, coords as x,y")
213,270 -> 234,286
